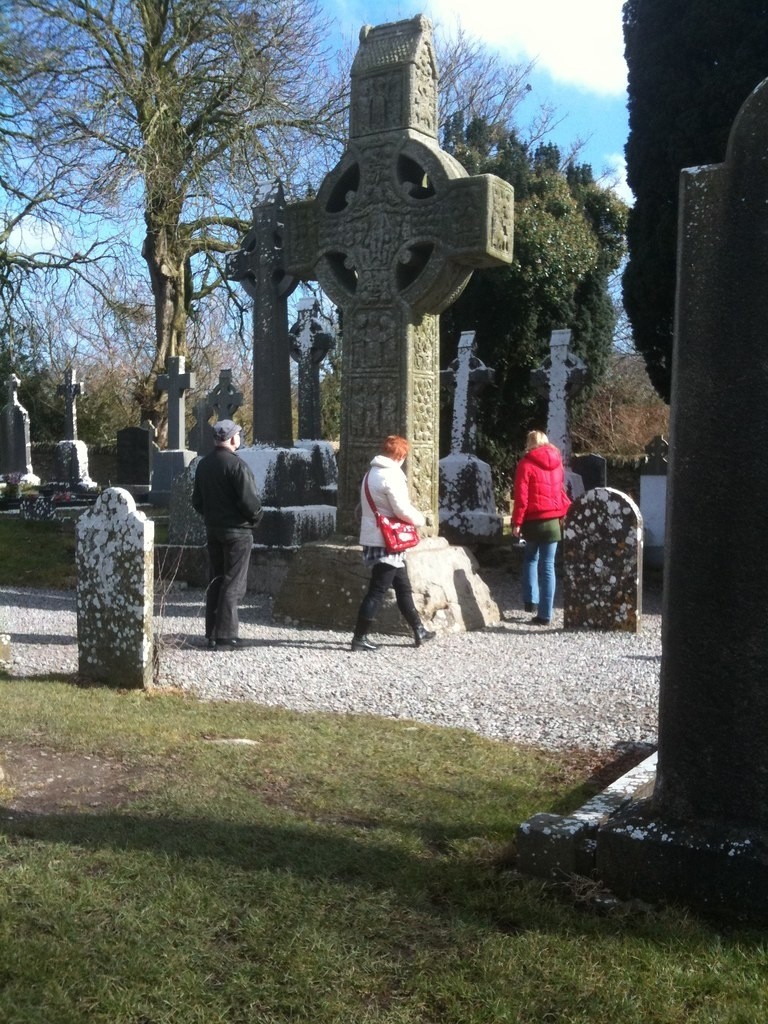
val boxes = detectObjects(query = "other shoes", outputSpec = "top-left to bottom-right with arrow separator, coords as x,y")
208,637 -> 217,647
216,636 -> 254,651
532,615 -> 550,626
526,602 -> 539,612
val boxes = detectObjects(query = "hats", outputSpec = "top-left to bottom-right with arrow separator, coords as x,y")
212,420 -> 242,441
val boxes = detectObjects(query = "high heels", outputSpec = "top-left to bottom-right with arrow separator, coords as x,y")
351,629 -> 379,652
412,624 -> 436,644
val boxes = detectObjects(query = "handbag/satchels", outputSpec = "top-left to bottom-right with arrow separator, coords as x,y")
374,515 -> 419,552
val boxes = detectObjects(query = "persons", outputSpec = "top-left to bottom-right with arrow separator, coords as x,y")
349,436 -> 436,649
512,431 -> 572,625
192,420 -> 264,647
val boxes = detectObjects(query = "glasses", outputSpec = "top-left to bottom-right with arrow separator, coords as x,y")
237,432 -> 242,438
